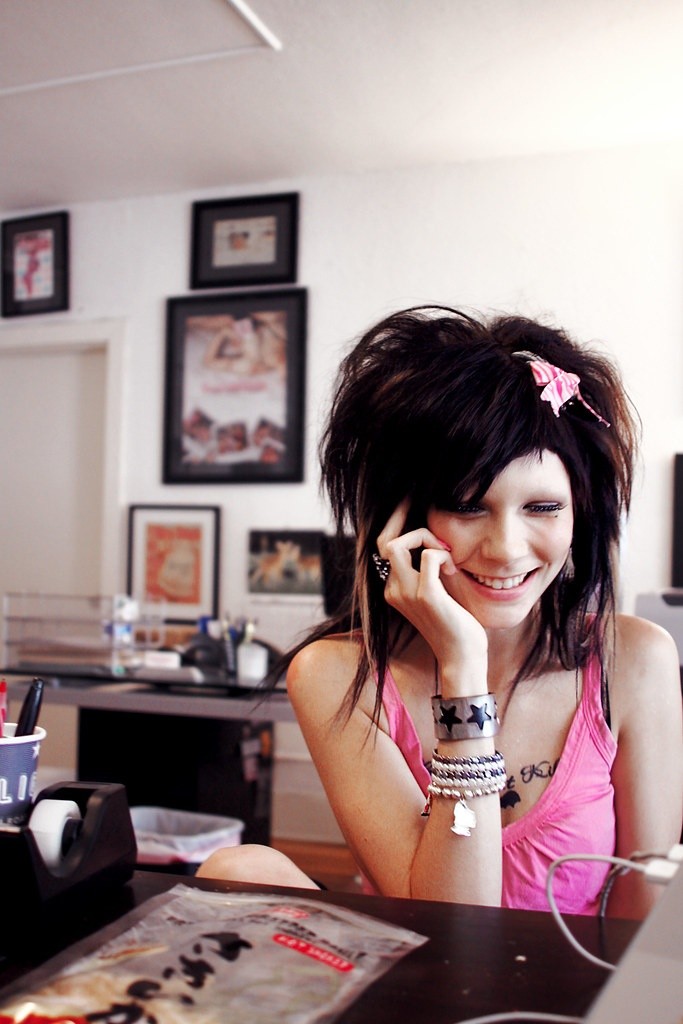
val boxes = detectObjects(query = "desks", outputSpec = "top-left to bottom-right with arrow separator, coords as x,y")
0,871 -> 642,1024
0,634 -> 299,846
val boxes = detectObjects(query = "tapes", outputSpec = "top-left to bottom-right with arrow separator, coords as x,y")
28,798 -> 83,876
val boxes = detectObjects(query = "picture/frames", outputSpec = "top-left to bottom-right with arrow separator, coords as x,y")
162,287 -> 308,483
127,504 -> 220,626
189,192 -> 299,290
0,211 -> 70,318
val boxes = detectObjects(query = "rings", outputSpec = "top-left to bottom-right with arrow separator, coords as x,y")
371,554 -> 390,583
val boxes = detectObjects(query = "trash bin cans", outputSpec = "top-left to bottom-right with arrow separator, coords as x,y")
130,806 -> 247,868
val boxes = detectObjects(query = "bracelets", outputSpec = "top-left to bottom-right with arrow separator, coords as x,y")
428,748 -> 509,838
431,693 -> 500,741
196,306 -> 683,921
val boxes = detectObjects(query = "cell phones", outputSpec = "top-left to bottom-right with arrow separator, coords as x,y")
402,503 -> 431,570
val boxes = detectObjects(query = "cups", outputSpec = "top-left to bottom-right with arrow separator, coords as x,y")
0,723 -> 46,827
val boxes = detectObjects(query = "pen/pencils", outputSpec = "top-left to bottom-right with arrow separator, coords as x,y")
14,675 -> 46,743
